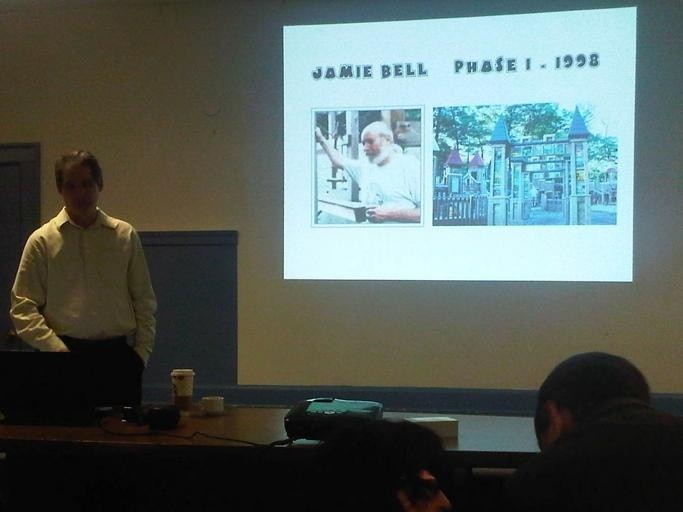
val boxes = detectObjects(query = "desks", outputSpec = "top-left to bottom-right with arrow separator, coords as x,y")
0,400 -> 683,487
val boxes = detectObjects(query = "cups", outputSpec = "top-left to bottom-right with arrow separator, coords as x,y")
171,367 -> 196,415
199,396 -> 226,416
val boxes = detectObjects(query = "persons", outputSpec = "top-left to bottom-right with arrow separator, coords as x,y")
473,350 -> 682,512
9,147 -> 158,411
314,118 -> 421,225
290,415 -> 460,512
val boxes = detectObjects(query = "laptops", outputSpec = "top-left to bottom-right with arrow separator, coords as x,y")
0,352 -> 138,427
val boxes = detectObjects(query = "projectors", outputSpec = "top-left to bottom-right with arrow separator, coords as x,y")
284,398 -> 382,443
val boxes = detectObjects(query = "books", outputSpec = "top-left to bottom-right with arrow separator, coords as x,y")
401,416 -> 459,444
